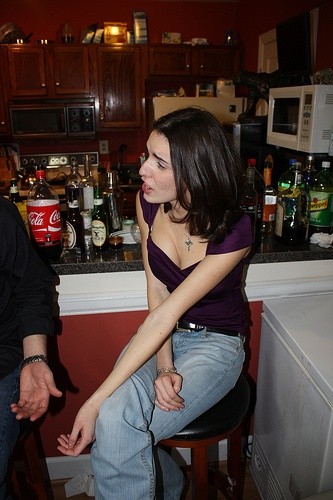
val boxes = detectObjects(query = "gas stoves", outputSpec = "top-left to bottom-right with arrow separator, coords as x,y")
16,152 -> 99,196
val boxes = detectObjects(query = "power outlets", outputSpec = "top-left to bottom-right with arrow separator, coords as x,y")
98,140 -> 109,154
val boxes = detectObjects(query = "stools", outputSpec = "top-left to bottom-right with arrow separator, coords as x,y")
157,374 -> 249,500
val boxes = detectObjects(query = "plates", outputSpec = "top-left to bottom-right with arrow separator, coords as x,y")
108,230 -> 140,246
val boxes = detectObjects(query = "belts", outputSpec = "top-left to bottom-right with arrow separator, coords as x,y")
176,321 -> 244,338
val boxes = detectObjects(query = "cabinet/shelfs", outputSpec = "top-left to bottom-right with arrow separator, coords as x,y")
0,43 -> 242,138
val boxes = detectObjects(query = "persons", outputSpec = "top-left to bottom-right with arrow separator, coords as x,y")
0,196 -> 63,482
57,108 -> 253,500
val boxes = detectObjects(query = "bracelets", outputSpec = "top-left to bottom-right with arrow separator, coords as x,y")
19,355 -> 49,370
157,368 -> 177,374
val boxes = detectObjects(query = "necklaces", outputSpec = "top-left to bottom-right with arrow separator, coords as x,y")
176,220 -> 193,251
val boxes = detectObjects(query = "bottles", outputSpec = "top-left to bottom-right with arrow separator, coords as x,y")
243,154 -> 333,241
61,21 -> 73,43
9,155 -> 123,259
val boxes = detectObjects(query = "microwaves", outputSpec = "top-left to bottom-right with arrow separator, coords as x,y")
264,85 -> 333,153
9,103 -> 95,139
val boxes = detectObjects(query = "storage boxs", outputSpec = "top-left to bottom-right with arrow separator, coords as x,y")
80,10 -> 207,44
